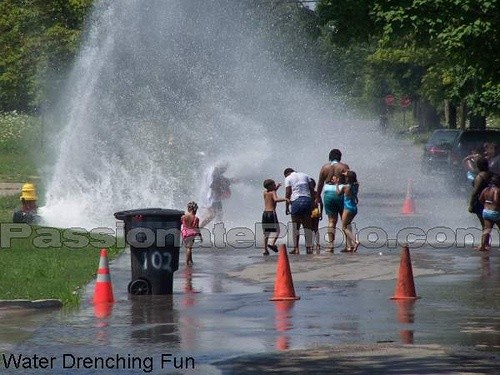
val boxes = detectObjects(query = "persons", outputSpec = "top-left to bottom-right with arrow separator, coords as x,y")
310,178 -> 323,250
468,158 -> 492,248
284,168 -> 314,254
464,146 -> 489,189
331,170 -> 360,252
316,149 -> 353,253
261,179 -> 291,255
181,201 -> 203,266
478,174 -> 500,252
199,160 -> 231,229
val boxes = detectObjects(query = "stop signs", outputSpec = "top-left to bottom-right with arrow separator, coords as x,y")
385,93 -> 395,108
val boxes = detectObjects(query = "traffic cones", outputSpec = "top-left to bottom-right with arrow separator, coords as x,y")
269,242 -> 303,302
272,303 -> 295,350
399,177 -> 419,216
391,302 -> 419,345
387,241 -> 426,300
92,246 -> 117,308
93,311 -> 114,346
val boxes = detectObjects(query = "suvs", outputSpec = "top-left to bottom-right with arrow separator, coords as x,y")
422,127 -> 461,174
448,127 -> 500,198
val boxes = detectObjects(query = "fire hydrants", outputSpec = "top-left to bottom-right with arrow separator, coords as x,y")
12,175 -> 42,223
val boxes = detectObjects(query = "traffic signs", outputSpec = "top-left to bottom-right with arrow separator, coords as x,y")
399,94 -> 411,109
